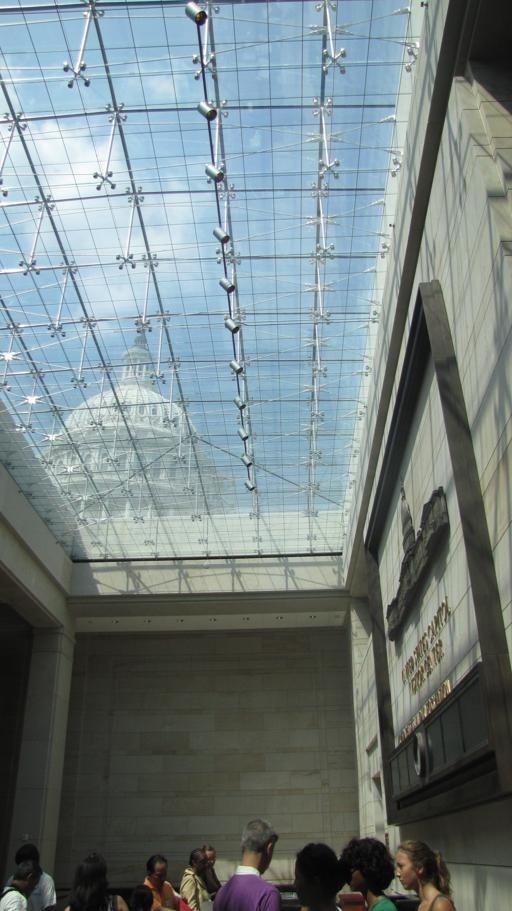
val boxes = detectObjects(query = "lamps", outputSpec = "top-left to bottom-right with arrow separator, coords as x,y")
176,3 -> 261,504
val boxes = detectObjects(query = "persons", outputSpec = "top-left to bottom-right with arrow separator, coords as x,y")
338,836 -> 398,911
129,884 -> 154,911
5,842 -> 58,911
394,838 -> 458,911
63,850 -> 129,911
292,841 -> 346,911
180,848 -> 211,911
144,854 -> 188,911
201,843 -> 223,901
0,859 -> 43,910
213,817 -> 282,911
